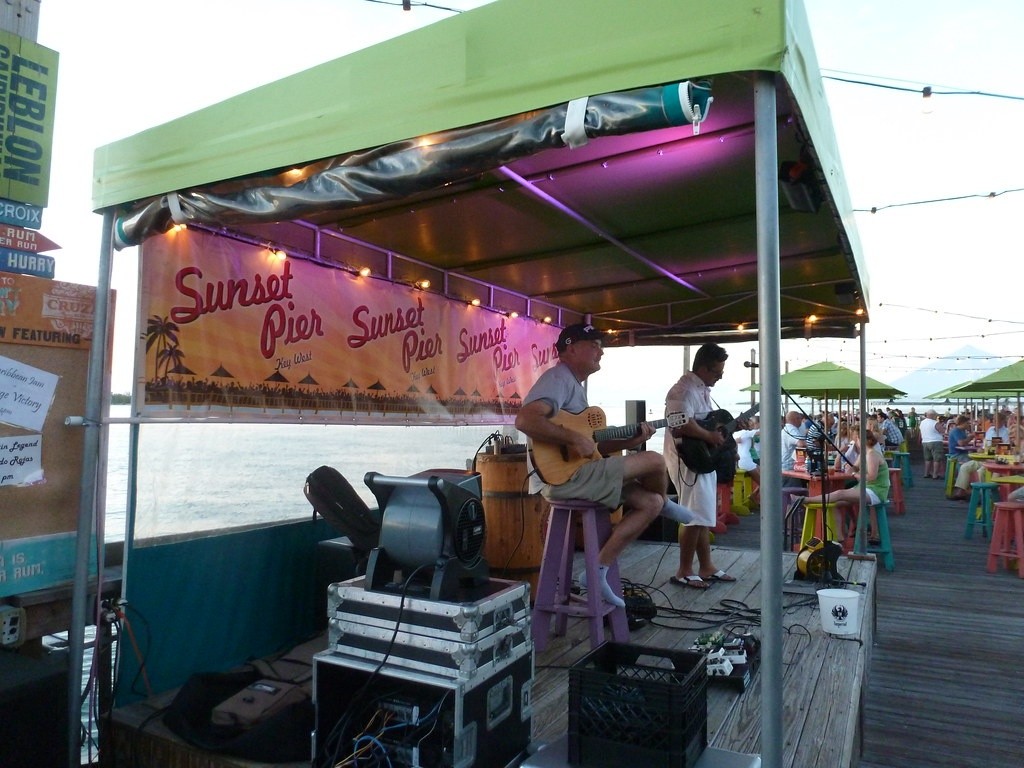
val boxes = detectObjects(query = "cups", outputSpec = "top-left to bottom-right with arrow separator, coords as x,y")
1013,456 -> 1020,465
828,466 -> 835,475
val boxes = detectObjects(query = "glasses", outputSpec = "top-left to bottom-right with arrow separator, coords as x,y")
708,367 -> 724,377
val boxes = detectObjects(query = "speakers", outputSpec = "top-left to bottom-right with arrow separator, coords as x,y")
626,400 -> 647,454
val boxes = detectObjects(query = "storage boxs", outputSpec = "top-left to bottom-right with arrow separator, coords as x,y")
311,574 -> 536,768
567,639 -> 709,768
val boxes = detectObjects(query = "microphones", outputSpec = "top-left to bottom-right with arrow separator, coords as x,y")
744,361 -> 759,368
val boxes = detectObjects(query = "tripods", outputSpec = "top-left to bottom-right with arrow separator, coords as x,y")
781,385 -> 867,589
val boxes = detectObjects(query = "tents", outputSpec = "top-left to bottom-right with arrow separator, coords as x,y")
63,0 -> 872,768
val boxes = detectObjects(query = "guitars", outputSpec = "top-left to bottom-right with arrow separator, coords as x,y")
526,405 -> 689,487
671,402 -> 759,474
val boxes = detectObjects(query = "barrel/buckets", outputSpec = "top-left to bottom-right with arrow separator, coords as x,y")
474,452 -> 623,608
816,589 -> 860,635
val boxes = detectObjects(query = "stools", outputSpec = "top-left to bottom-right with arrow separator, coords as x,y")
675,428 -> 1024,579
530,499 -> 631,653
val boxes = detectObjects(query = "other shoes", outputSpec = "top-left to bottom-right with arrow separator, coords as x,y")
747,494 -> 759,505
947,489 -> 968,500
790,494 -> 799,503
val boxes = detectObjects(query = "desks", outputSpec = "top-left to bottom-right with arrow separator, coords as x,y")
983,463 -> 1024,542
781,470 -> 856,556
968,453 -> 1015,522
989,475 -> 1024,570
954,445 -> 980,460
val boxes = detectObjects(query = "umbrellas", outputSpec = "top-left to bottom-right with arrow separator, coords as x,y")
740,359 -> 907,471
922,359 -> 1024,458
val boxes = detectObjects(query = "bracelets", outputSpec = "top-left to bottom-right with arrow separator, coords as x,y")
851,472 -> 856,477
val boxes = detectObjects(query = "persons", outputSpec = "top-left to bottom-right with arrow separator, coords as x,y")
919,409 -> 945,480
732,407 -> 906,504
790,429 -> 890,543
662,343 -> 749,588
937,404 -> 1024,570
514,324 -> 696,609
907,407 -> 918,439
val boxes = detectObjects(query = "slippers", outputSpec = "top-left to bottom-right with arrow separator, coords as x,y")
702,570 -> 736,581
868,537 -> 880,541
924,475 -> 931,477
933,475 -> 944,480
669,574 -> 710,588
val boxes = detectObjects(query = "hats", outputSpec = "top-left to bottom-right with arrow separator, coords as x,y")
555,324 -> 608,351
948,419 -> 956,423
957,416 -> 970,424
